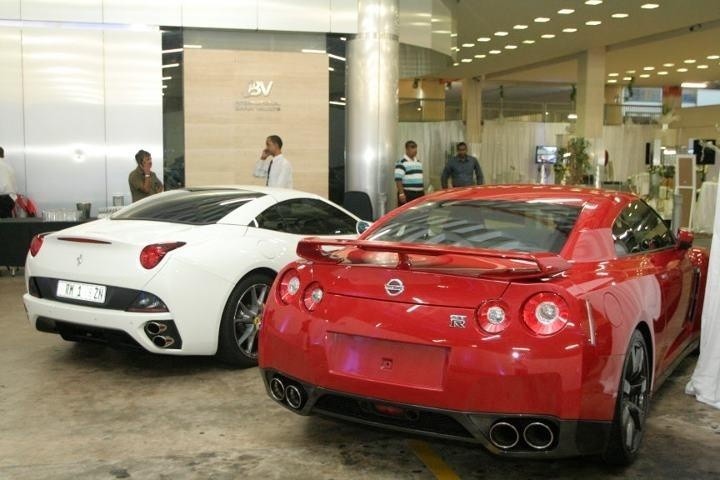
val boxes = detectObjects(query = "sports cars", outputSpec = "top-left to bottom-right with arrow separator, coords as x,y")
22,184 -> 373,368
253,183 -> 711,467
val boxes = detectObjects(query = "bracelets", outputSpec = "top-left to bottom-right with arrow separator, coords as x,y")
145,174 -> 151,178
399,193 -> 405,197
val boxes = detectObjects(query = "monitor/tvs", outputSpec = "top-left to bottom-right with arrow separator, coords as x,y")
535,145 -> 558,164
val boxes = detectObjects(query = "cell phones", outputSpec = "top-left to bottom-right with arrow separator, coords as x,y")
140,159 -> 146,166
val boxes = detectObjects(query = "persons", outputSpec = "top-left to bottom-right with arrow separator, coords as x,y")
441,143 -> 484,191
0,146 -> 16,207
394,140 -> 426,207
253,135 -> 293,190
129,149 -> 165,205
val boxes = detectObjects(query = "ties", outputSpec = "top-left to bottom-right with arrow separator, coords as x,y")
266,160 -> 273,187
